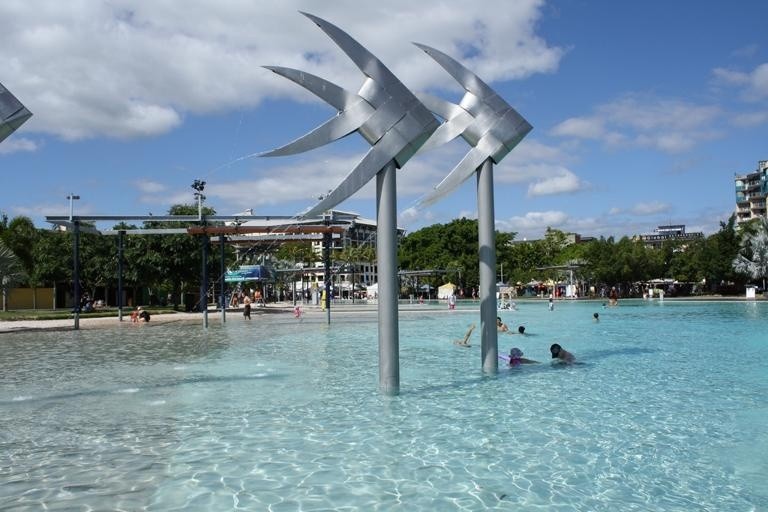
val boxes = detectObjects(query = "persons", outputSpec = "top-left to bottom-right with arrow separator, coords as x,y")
417,293 -> 428,307
232,293 -> 239,308
136,305 -> 150,322
320,289 -> 329,312
294,306 -> 304,319
549,343 -> 575,362
441,282 -> 638,313
495,317 -> 510,333
79,290 -> 108,313
253,287 -> 263,305
129,311 -> 140,322
454,323 -> 478,350
241,291 -> 252,320
591,312 -> 600,320
509,325 -> 530,339
509,348 -> 525,366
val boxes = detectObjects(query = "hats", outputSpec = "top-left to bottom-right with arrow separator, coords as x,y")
550,343 -> 561,358
508,347 -> 523,358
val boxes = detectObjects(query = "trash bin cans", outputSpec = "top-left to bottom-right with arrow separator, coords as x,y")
746,285 -> 756,297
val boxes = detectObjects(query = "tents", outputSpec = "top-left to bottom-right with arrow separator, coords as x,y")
434,281 -> 464,302
